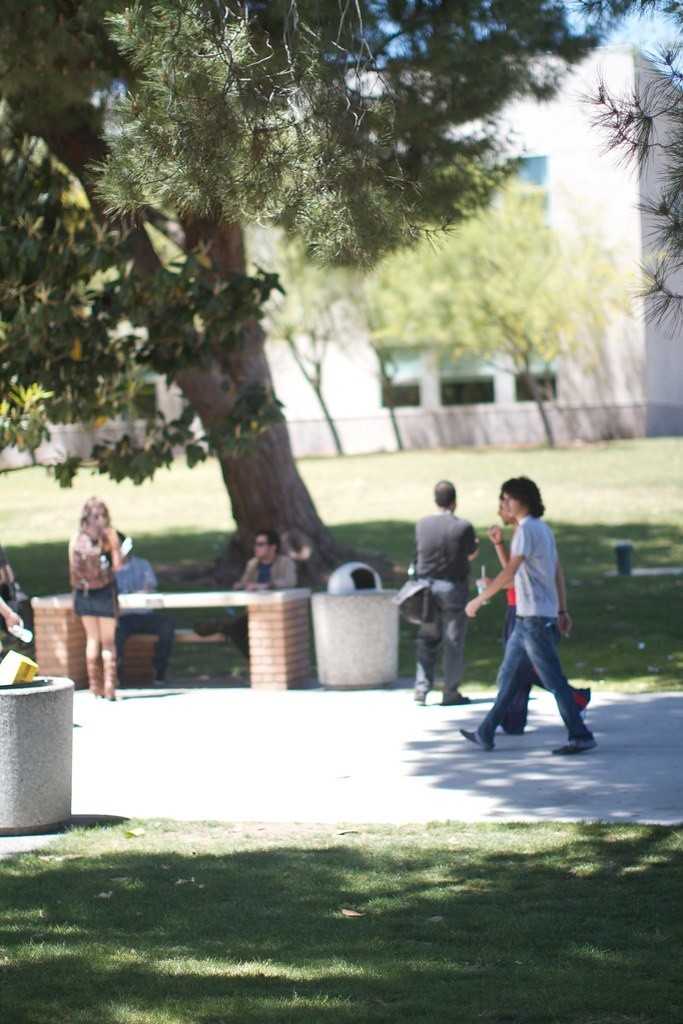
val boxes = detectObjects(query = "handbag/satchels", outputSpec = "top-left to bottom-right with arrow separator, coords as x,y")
391,579 -> 434,625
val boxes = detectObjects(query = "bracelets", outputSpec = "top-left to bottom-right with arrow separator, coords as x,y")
558,610 -> 568,615
493,540 -> 504,546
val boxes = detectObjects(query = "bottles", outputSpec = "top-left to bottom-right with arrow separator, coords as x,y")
100,556 -> 110,571
12,625 -> 33,642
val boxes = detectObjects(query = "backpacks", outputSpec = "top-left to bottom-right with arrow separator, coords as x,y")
70,526 -> 113,597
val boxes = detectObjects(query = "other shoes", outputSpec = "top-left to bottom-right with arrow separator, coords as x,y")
442,695 -> 472,704
551,745 -> 588,755
460,728 -> 477,745
416,691 -> 427,701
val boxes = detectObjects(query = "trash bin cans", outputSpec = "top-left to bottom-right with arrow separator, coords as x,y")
310,563 -> 400,688
1,676 -> 75,836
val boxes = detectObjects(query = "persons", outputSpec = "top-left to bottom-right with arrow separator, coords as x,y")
69,497 -> 121,700
100,532 -> 174,685
487,492 -> 591,734
0,545 -> 24,663
413,481 -> 479,706
460,477 -> 597,754
229,531 -> 297,679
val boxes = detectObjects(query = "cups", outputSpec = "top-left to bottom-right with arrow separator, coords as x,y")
478,578 -> 491,606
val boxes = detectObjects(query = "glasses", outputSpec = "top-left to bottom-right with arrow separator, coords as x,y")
253,540 -> 268,546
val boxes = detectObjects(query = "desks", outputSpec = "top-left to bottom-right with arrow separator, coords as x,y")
30,587 -> 312,692
